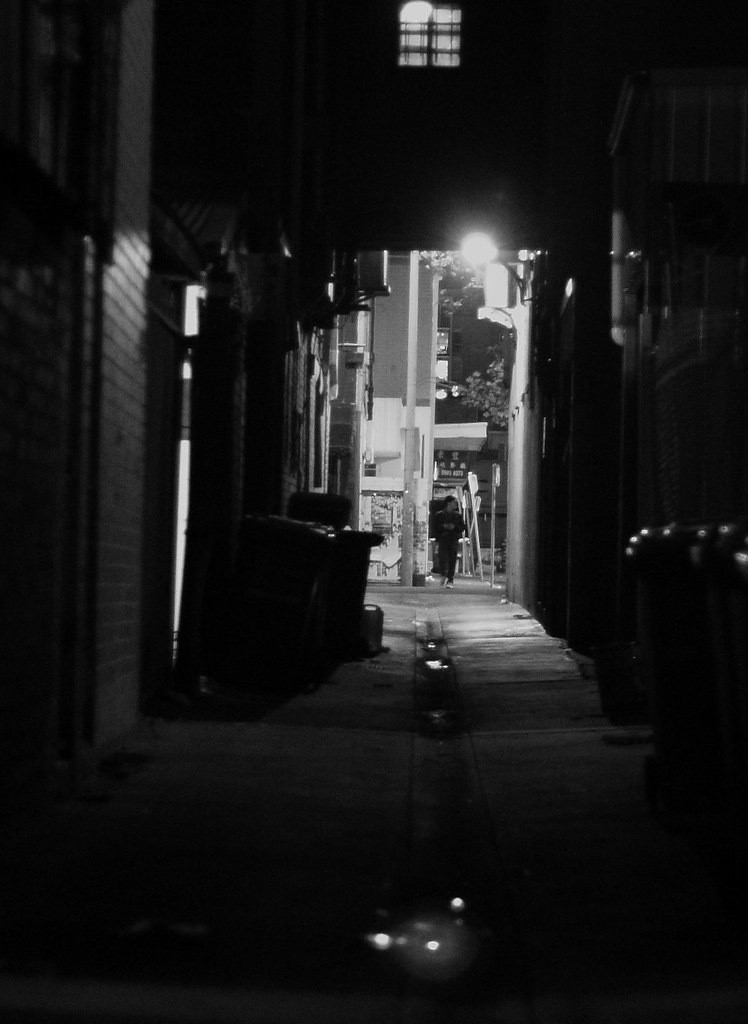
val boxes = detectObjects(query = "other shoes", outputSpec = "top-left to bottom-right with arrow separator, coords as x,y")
446,580 -> 453,588
440,577 -> 446,584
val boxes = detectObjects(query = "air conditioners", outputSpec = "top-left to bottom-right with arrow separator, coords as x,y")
356,250 -> 391,297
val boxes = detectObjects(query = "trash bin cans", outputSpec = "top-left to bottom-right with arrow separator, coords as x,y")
213,491 -> 383,702
624,512 -> 748,814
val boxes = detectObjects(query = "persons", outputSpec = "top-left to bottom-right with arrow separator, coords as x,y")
432,494 -> 466,589
